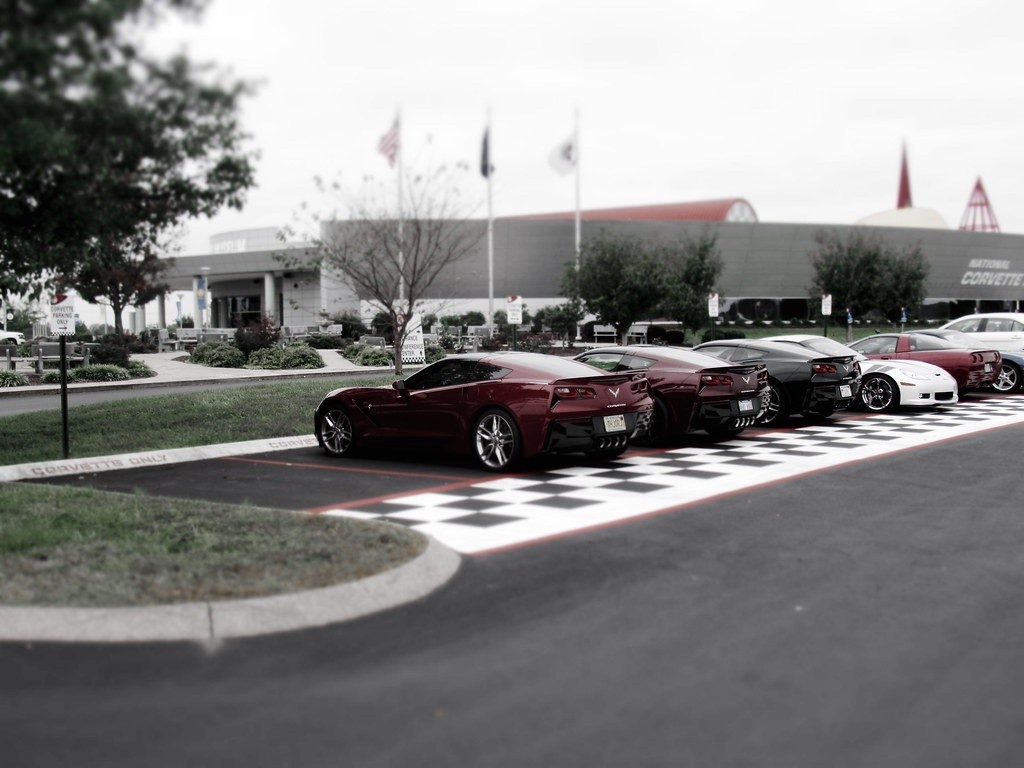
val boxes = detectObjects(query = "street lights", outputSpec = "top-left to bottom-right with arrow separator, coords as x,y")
201,265 -> 212,335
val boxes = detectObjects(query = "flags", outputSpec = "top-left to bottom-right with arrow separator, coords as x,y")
480,126 -> 495,181
379,116 -> 400,166
560,131 -> 579,172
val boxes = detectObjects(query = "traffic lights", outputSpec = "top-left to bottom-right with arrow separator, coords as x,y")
6,309 -> 16,323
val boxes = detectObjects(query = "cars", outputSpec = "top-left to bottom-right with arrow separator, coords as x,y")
0,331 -> 26,346
568,348 -> 771,449
313,351 -> 654,471
693,312 -> 1024,423
